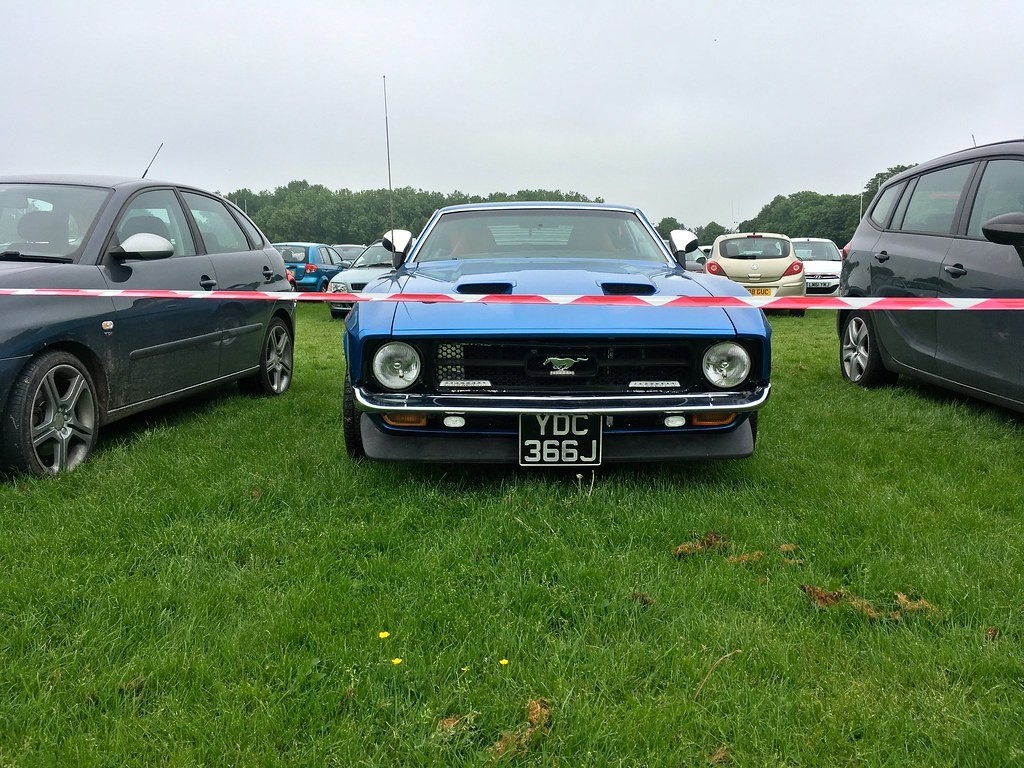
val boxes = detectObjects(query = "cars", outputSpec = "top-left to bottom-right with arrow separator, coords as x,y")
773,237 -> 844,297
660,240 -> 710,274
268,242 -> 346,303
342,200 -> 774,466
0,172 -> 297,480
836,138 -> 1024,416
696,230 -> 807,317
325,238 -> 419,318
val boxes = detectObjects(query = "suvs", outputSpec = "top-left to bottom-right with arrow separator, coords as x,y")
328,243 -> 367,268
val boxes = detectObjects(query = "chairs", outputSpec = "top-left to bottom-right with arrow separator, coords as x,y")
446,223 -> 497,255
283,250 -> 298,262
568,222 -> 616,251
726,243 -> 739,256
115,215 -> 171,252
762,244 -> 780,255
811,247 -> 829,259
6,212 -> 67,256
